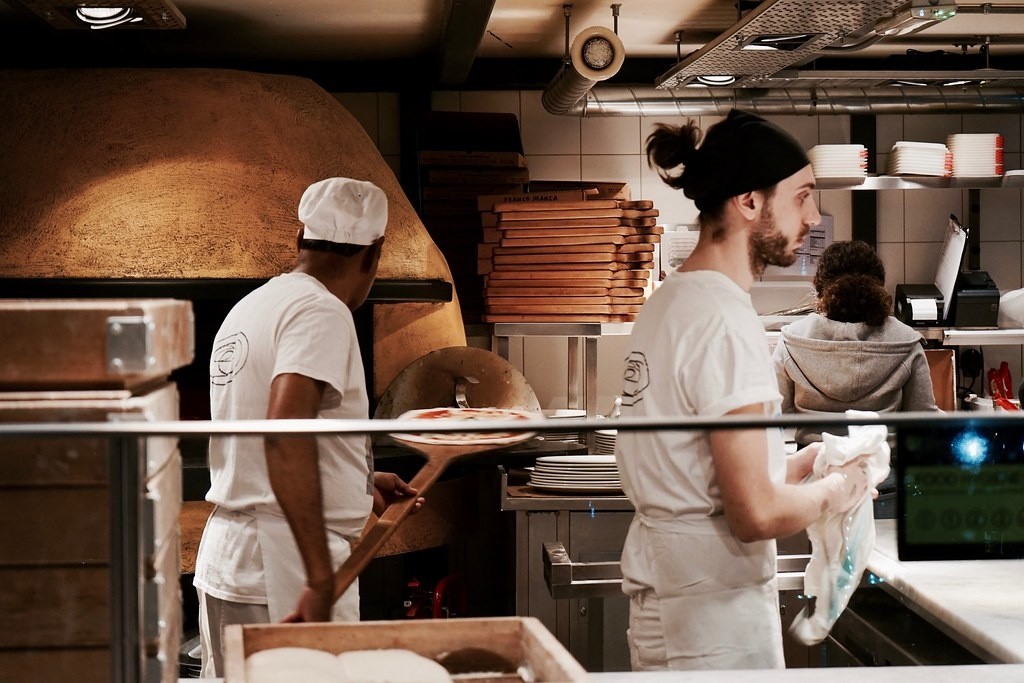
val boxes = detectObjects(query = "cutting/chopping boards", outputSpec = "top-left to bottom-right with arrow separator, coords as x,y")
481,200 -> 663,324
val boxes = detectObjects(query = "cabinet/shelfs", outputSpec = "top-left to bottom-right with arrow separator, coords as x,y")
494,169 -> 1024,346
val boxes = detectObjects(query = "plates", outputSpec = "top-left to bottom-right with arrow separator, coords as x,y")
527,455 -> 622,492
593,430 -> 617,455
543,410 -> 586,418
886,134 -> 1004,177
545,432 -> 585,444
806,145 -> 868,179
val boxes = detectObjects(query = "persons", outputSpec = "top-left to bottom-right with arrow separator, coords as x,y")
192,177 -> 424,682
778,239 -> 945,513
616,107 -> 879,669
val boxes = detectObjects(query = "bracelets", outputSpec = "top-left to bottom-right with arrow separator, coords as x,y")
308,573 -> 336,588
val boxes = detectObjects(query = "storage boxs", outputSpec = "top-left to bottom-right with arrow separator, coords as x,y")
0,299 -> 196,389
223,617 -> 600,683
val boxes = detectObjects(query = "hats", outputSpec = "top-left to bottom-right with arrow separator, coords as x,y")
297,177 -> 388,246
